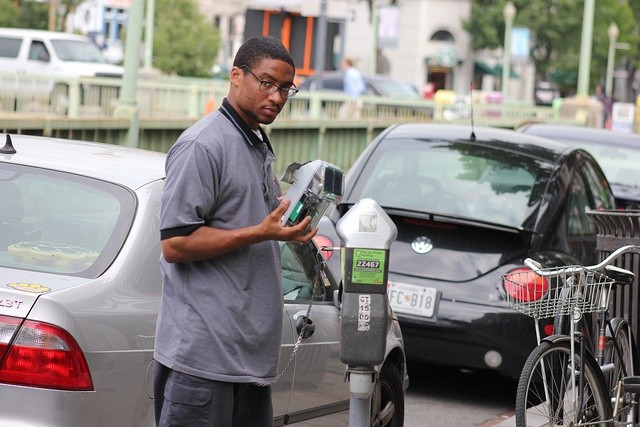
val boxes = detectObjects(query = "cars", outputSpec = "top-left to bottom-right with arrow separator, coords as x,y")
465,124 -> 640,216
329,120 -> 616,383
1,132 -> 410,425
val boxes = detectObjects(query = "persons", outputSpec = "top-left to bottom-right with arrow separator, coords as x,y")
338,57 -> 367,120
153,34 -> 321,427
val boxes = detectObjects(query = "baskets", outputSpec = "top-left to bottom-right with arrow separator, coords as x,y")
500,265 -> 616,320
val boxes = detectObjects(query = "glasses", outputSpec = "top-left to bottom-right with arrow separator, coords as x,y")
240,65 -> 298,98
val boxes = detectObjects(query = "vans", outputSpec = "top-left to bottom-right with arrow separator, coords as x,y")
0,26 -> 126,115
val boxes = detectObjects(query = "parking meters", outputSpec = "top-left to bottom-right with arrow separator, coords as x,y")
333,195 -> 398,426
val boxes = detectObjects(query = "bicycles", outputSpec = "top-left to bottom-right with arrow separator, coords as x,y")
501,245 -> 640,426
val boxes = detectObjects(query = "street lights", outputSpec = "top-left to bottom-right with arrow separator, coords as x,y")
502,0 -> 517,126
604,20 -> 620,97
